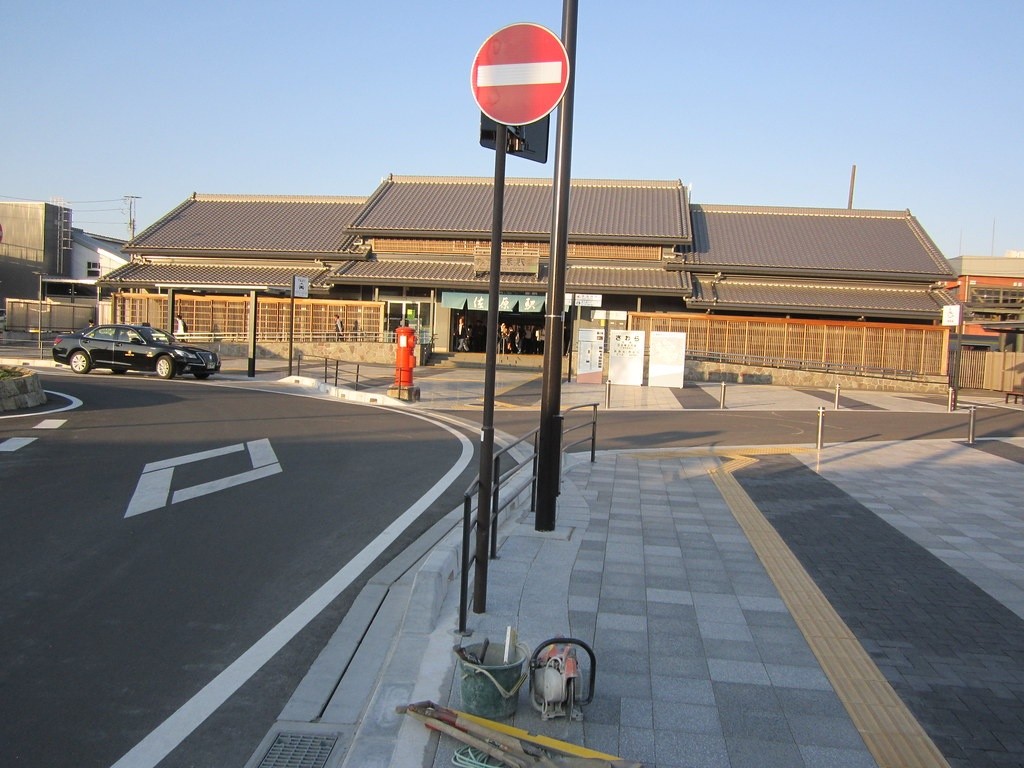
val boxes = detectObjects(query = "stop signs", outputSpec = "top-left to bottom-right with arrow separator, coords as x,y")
470,23 -> 571,126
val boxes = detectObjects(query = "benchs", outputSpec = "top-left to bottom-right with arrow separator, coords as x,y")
1004,391 -> 1024,405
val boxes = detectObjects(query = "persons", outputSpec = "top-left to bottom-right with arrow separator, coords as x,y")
499,322 -> 544,354
87,320 -> 95,327
334,315 -> 343,336
453,316 -> 470,352
175,315 -> 187,334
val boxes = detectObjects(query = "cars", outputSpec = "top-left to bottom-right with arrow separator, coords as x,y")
52,323 -> 221,381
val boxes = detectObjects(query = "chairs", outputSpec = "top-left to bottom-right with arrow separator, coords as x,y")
121,333 -> 129,341
